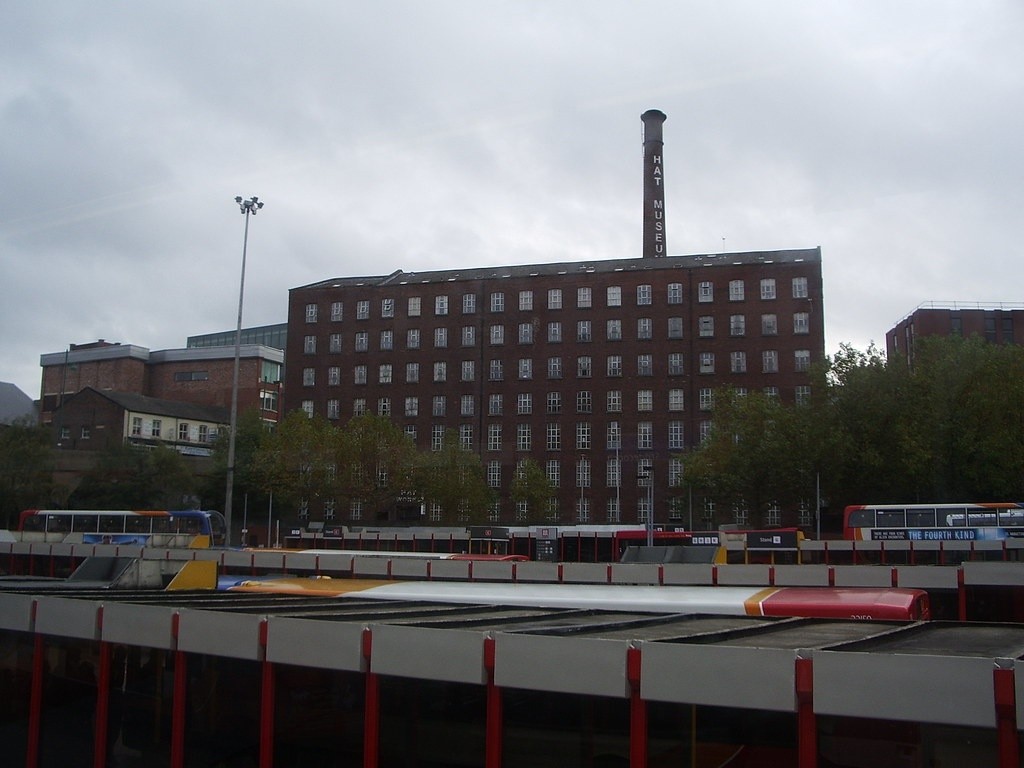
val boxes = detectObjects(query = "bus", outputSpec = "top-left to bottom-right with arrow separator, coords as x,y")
842,499 -> 1024,542
19,511 -> 227,546
614,527 -> 799,563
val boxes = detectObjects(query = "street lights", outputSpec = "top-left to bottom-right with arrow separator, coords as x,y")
223,193 -> 264,545
